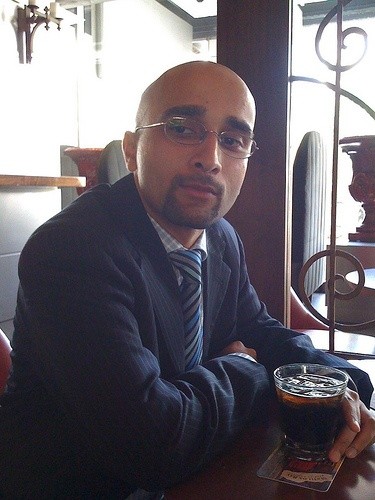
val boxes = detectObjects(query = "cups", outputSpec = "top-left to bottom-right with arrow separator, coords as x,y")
273,362 -> 348,462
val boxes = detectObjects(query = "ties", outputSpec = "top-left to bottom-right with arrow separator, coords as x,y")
168,249 -> 203,372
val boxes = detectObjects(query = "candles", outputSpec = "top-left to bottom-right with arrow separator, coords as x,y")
49,2 -> 59,14
29,0 -> 36,4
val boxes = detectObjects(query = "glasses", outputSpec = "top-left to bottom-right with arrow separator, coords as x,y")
135,116 -> 260,159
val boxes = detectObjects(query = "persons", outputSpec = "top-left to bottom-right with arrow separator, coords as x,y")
0,60 -> 375,498
